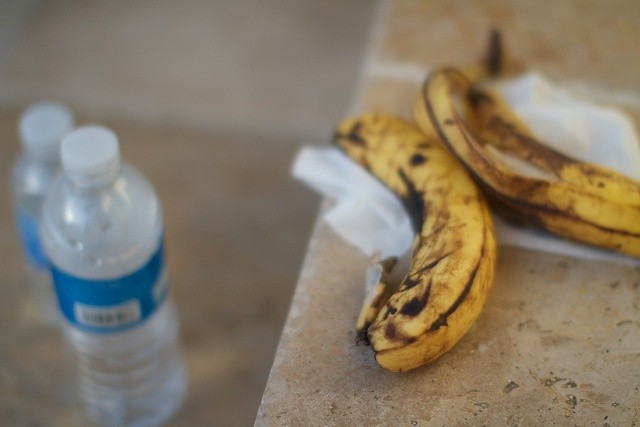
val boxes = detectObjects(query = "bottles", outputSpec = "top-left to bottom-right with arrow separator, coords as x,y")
13,103 -> 75,272
41,124 -> 189,426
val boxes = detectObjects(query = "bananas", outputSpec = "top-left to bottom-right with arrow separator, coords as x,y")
332,111 -> 497,373
417,64 -> 640,261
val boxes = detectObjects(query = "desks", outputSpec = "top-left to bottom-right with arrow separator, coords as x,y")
253,1 -> 640,426
